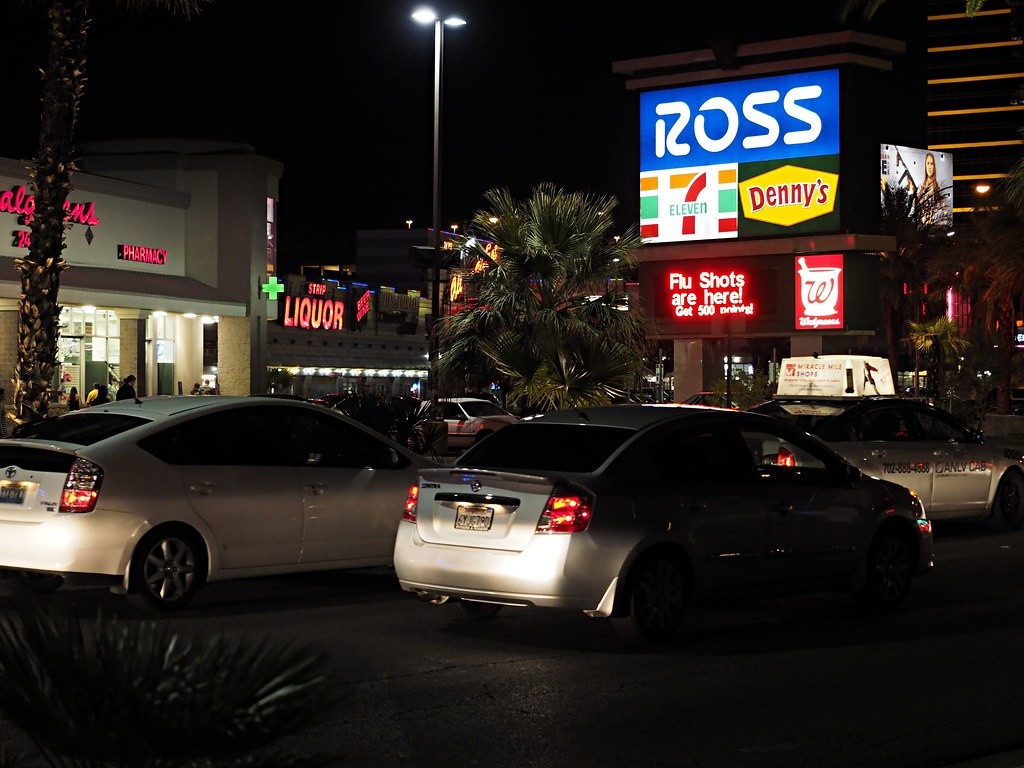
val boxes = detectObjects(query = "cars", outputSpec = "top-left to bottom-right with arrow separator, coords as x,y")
905,387 -> 1024,416
394,404 -> 931,648
322,391 -> 520,450
741,352 -> 1024,531
0,396 -> 451,613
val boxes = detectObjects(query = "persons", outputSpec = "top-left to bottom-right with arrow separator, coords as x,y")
0,389 -> 7,436
919,153 -> 948,226
191,380 -> 214,395
68,388 -> 82,411
86,375 -> 136,407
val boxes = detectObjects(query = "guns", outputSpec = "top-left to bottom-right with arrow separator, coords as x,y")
894,145 -> 918,192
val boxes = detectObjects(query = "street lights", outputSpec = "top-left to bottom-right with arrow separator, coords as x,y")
411,7 -> 466,422
